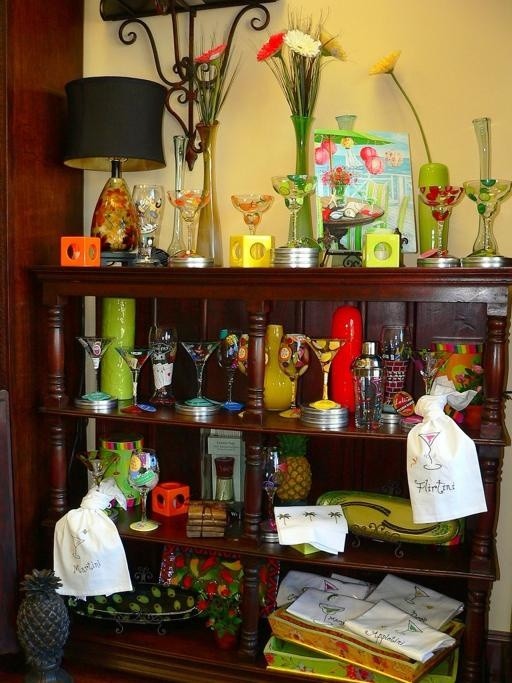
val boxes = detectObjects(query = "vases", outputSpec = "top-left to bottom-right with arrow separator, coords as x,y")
471,116 -> 498,253
417,163 -> 450,256
168,121 -> 224,267
465,404 -> 483,432
289,115 -> 314,247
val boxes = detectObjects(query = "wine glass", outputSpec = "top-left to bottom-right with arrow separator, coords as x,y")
465,179 -> 512,256
259,446 -> 282,532
306,340 -> 346,410
78,335 -> 115,401
78,450 -> 119,519
166,187 -> 212,259
181,340 -> 220,405
271,174 -> 319,249
418,185 -> 467,259
409,350 -> 453,394
115,348 -> 153,415
219,330 -> 243,406
418,431 -> 442,472
277,332 -> 310,419
128,448 -> 161,532
355,368 -> 383,428
70,535 -> 85,560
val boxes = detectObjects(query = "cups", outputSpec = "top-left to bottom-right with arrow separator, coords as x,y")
381,326 -> 411,415
149,329 -> 177,406
132,185 -> 165,264
231,193 -> 274,234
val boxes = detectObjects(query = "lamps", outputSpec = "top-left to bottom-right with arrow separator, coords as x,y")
61,76 -> 167,254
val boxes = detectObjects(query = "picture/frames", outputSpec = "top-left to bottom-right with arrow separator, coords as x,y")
158,546 -> 281,617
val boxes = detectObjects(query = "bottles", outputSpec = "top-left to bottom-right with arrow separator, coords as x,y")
166,134 -> 189,255
214,456 -> 236,504
349,342 -> 381,376
261,325 -> 293,412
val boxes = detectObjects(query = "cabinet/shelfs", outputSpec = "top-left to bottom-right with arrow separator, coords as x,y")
28,259 -> 510,680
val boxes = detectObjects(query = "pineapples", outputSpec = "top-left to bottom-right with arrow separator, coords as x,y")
15,568 -> 69,667
272,432 -> 313,502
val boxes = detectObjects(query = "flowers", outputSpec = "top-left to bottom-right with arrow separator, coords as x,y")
368,48 -> 432,164
248,3 -> 346,139
185,24 -> 243,125
455,363 -> 488,405
